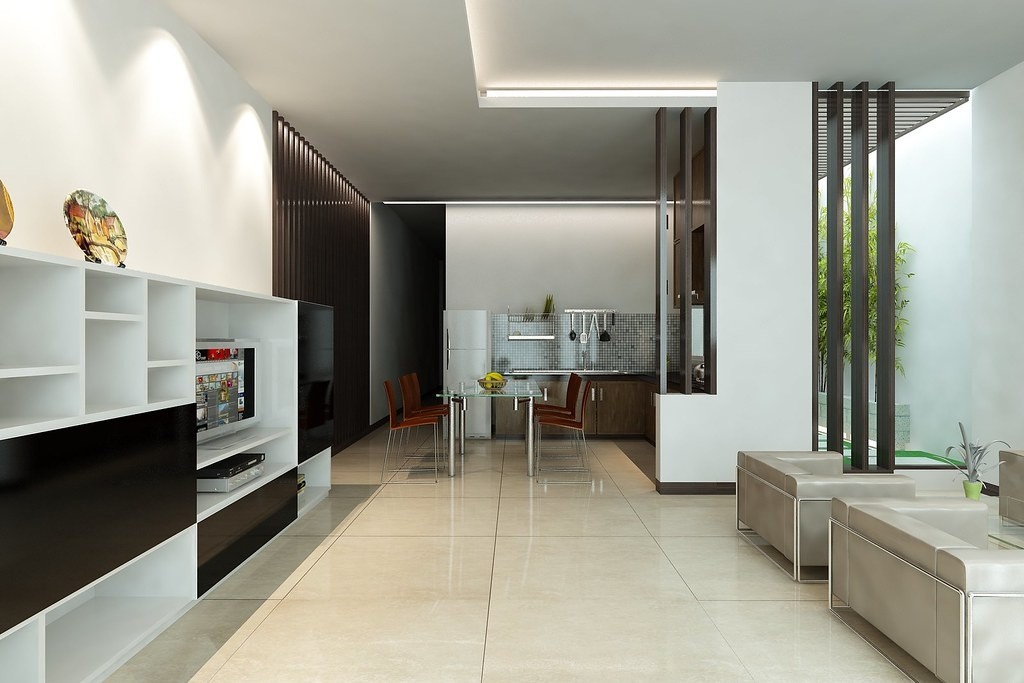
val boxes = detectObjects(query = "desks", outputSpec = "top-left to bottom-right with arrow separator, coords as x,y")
436,379 -> 543,477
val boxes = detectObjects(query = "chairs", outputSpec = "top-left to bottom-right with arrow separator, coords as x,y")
525,372 -> 591,484
380,373 -> 448,484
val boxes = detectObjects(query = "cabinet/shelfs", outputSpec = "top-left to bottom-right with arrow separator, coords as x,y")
506,306 -> 555,341
495,380 -> 681,448
673,146 -> 705,308
0,244 -> 333,683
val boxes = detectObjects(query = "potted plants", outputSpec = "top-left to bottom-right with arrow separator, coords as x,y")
932,422 -> 1011,500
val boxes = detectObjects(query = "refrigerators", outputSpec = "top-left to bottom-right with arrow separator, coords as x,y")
442,309 -> 492,440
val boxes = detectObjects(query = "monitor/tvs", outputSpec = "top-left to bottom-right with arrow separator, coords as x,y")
196,337 -> 262,449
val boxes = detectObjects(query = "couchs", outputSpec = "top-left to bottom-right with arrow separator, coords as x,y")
736,451 -> 917,584
998,450 -> 1024,530
828,495 -> 1024,682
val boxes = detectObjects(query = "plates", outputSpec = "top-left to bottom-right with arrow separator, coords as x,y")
64,189 -> 128,266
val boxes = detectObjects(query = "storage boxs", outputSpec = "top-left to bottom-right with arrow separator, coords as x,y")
297,474 -> 305,494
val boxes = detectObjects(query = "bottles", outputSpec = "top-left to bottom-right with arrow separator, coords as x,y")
667,353 -> 671,373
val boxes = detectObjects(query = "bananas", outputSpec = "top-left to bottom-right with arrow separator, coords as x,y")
487,373 -> 503,381
486,375 -> 492,388
